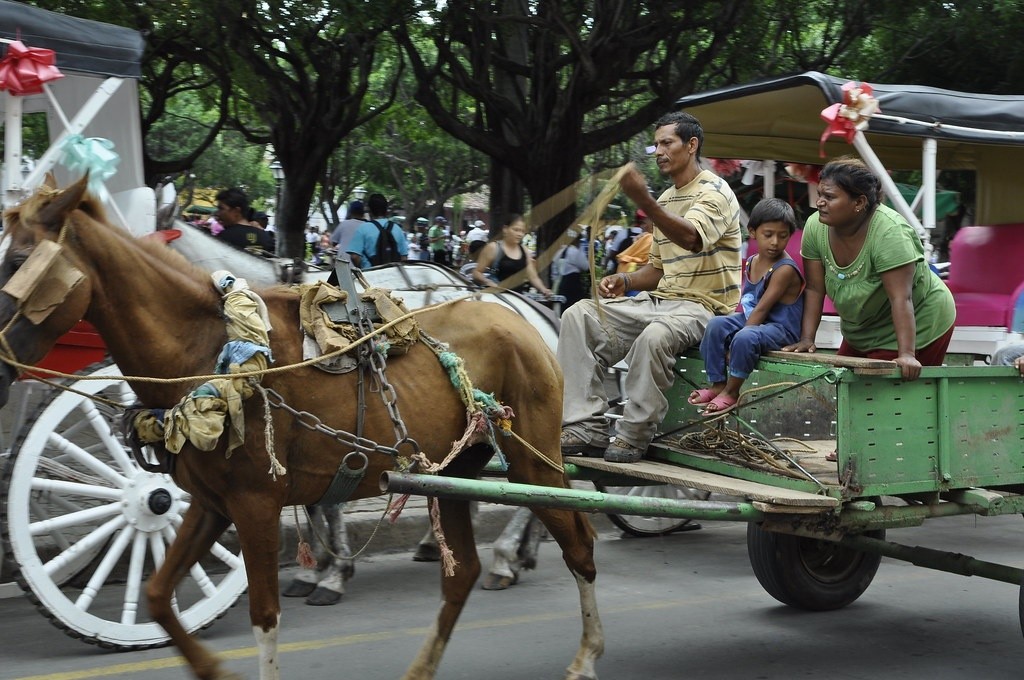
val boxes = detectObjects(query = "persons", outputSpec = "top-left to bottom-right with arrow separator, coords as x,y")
551,111 -> 742,462
183,184 -> 277,259
779,157 -> 957,378
305,189 -> 554,298
551,208 -> 655,319
688,197 -> 807,418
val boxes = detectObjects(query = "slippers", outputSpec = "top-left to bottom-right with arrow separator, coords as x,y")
687,389 -> 718,406
701,394 -> 742,417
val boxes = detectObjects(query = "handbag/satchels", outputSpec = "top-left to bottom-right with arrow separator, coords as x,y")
487,239 -> 502,284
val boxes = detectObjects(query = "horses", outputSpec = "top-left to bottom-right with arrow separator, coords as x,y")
1,168 -> 607,680
163,221 -> 569,609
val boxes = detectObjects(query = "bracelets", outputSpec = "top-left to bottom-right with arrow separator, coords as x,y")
620,272 -> 632,293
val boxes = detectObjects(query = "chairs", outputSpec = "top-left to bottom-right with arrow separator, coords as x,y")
742,227 -> 848,348
938,223 -> 1023,350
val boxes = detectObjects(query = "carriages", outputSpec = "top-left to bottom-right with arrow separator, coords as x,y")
0,72 -> 1024,680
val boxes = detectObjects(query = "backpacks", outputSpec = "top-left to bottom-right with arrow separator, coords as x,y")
363,219 -> 401,266
612,228 -> 641,274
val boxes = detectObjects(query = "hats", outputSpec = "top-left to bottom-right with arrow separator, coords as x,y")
435,216 -> 446,221
636,208 -> 649,219
254,212 -> 271,219
475,220 -> 486,227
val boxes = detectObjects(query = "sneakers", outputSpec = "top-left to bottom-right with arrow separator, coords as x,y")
604,437 -> 647,463
561,428 -> 607,458
